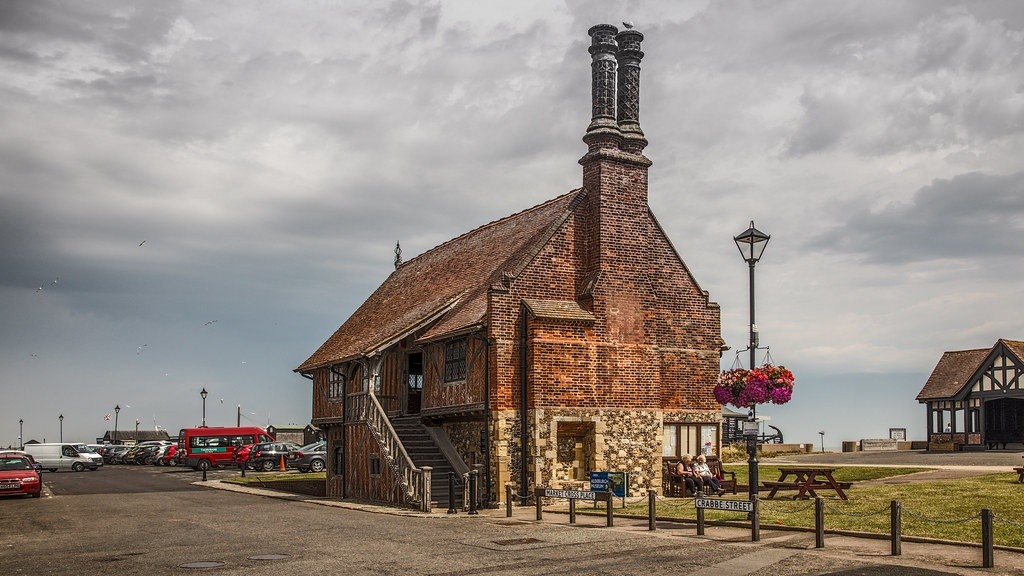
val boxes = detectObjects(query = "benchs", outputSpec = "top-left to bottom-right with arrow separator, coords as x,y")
762,481 -> 801,489
815,479 -> 852,489
667,461 -> 737,498
1013,468 -> 1024,474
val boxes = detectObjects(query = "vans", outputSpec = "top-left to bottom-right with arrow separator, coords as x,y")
23,442 -> 103,472
178,427 -> 275,473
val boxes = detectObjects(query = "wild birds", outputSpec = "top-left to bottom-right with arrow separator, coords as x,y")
139,240 -> 145,246
51,278 -> 59,285
204,319 -> 217,326
35,284 -> 44,293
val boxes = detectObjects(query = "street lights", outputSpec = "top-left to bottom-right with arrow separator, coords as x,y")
59,414 -> 64,444
19,418 -> 25,451
199,387 -> 209,428
114,405 -> 120,446
733,220 -> 771,542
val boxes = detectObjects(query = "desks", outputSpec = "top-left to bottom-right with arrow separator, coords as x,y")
766,468 -> 848,501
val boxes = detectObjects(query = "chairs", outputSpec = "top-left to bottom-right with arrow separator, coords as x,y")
0,462 -> 25,469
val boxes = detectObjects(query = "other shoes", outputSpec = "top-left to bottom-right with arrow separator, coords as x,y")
702,493 -> 707,497
719,490 -> 726,496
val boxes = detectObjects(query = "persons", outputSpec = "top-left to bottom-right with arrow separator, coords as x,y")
945,424 -> 951,433
674,454 -> 704,498
693,455 -> 726,496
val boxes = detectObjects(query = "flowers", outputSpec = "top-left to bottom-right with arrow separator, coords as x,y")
713,363 -> 797,409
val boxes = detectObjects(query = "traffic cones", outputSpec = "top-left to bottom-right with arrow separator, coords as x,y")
279,452 -> 286,472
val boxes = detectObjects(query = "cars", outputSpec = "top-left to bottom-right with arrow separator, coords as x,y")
0,450 -> 42,489
232,443 -> 257,470
0,455 -> 41,497
88,442 -> 132,464
248,441 -> 302,470
296,440 -> 327,473
123,441 -> 182,466
